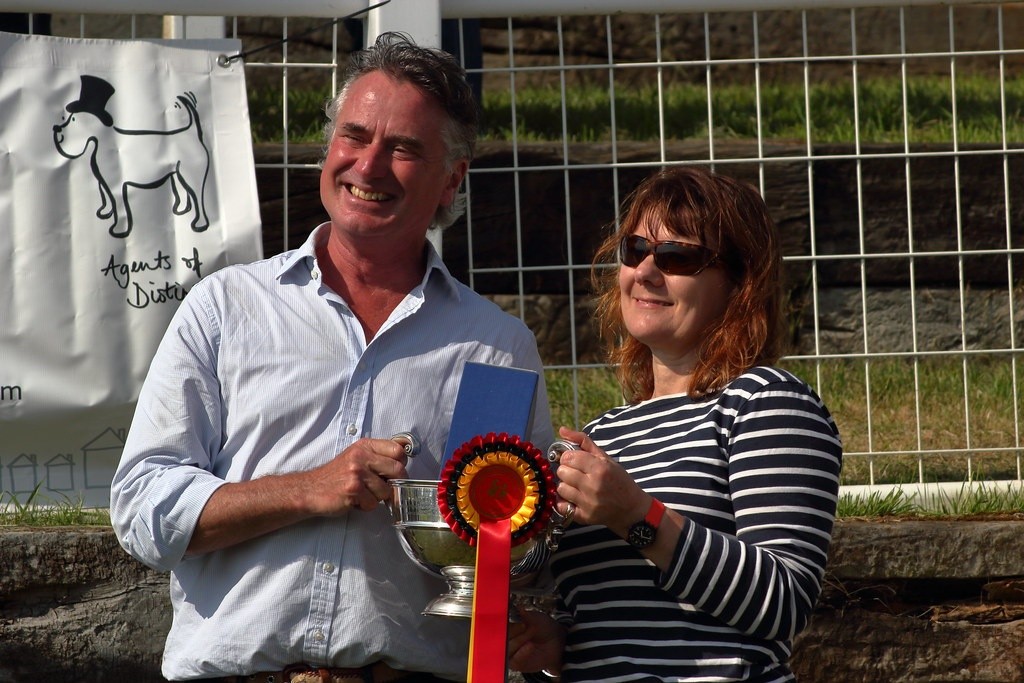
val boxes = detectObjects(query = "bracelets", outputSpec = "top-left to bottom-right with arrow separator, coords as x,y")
542,670 -> 561,678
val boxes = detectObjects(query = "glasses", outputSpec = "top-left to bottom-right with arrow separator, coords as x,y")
619,233 -> 721,276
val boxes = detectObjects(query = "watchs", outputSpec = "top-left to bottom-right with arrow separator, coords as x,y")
625,498 -> 664,549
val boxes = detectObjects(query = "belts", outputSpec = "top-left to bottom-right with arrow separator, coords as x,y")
193,661 -> 433,683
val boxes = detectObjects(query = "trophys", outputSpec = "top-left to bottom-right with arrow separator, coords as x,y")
382,433 -> 581,683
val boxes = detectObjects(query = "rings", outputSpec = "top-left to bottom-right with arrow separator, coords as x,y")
565,505 -> 574,518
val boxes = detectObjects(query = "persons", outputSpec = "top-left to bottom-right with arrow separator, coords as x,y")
110,30 -> 561,683
505,169 -> 843,682
